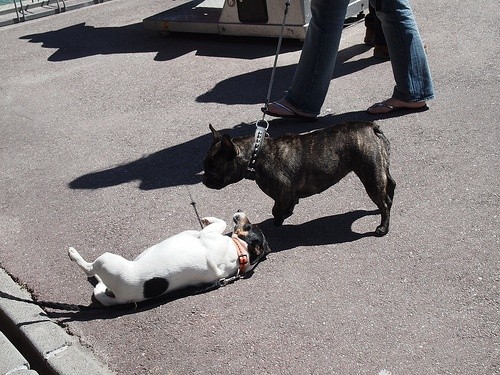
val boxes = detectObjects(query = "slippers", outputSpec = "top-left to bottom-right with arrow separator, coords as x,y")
366,97 -> 427,115
261,101 -> 317,120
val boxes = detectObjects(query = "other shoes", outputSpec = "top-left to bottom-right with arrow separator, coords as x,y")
364,28 -> 427,59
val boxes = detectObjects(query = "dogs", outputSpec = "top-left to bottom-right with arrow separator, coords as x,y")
66,208 -> 271,310
198,120 -> 396,240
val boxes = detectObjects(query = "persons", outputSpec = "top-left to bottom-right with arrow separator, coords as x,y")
261,0 -> 435,122
